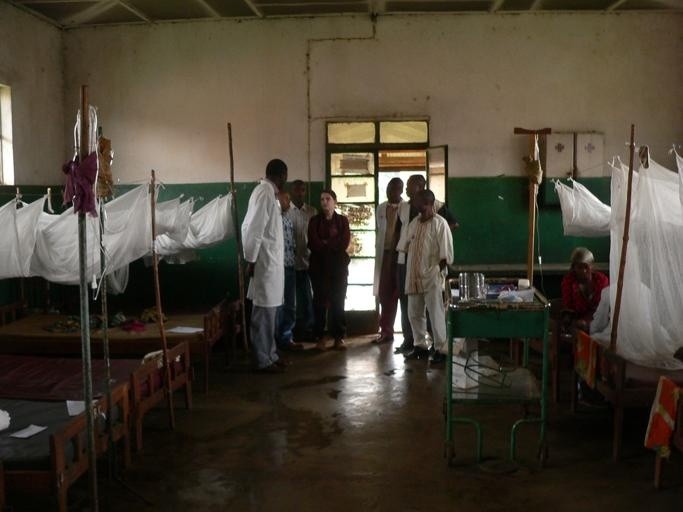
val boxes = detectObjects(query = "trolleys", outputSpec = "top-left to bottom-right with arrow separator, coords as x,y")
444,271 -> 549,477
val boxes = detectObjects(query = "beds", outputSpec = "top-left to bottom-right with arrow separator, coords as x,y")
0,339 -> 192,452
654,377 -> 683,491
569,325 -> 683,465
0,291 -> 238,371
1,382 -> 130,511
520,319 -> 573,431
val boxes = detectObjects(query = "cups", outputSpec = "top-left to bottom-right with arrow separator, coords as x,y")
517,278 -> 530,291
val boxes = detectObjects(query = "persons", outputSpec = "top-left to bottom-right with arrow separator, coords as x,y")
291,179 -> 319,342
562,247 -> 609,333
370,177 -> 403,345
398,190 -> 455,368
307,188 -> 347,351
395,175 -> 458,359
277,188 -> 304,352
241,158 -> 293,374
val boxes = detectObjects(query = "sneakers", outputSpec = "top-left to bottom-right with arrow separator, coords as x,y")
373,335 -> 447,365
256,337 -> 349,374
